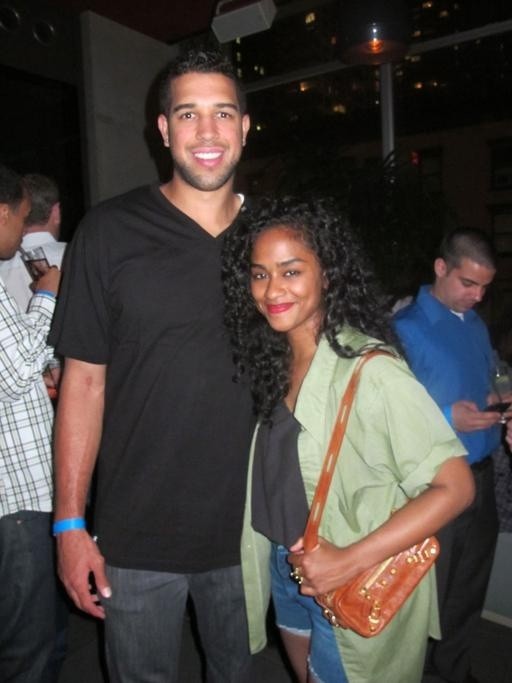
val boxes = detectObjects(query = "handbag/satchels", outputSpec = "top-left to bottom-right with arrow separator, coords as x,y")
301,349 -> 441,638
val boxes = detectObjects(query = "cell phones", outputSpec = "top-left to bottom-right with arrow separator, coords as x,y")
482,402 -> 511,415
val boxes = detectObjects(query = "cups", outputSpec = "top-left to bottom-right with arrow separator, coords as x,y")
20,246 -> 52,282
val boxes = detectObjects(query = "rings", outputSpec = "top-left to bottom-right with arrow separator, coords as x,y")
288,567 -> 304,584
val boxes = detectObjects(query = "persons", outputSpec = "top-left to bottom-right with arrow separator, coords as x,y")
36,43 -> 282,682
1,166 -> 74,320
221,187 -> 478,683
0,154 -> 70,681
381,220 -> 512,683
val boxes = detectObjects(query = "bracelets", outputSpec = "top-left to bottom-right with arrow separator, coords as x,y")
52,517 -> 87,535
